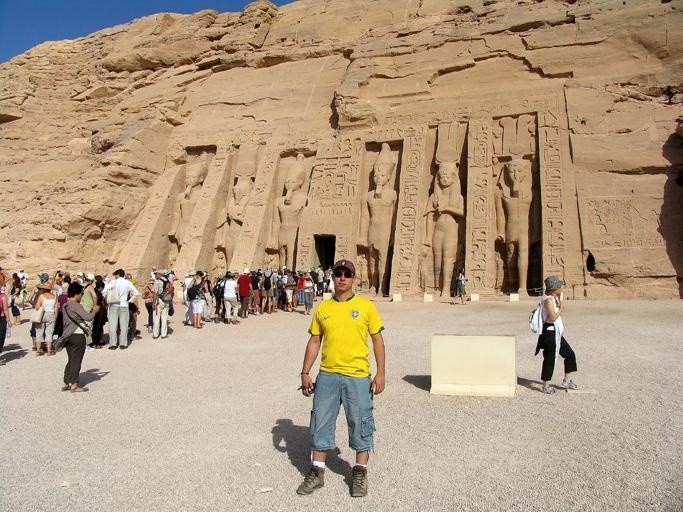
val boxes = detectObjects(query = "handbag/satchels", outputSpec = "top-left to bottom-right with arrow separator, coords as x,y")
188,277 -> 204,300
15,297 -> 24,306
83,326 -> 93,337
455,279 -> 462,287
30,308 -> 45,324
220,280 -> 227,298
107,279 -> 120,304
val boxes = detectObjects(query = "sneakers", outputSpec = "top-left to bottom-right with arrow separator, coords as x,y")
352,465 -> 368,497
296,465 -> 325,495
32,342 -> 56,355
182,306 -> 312,329
561,379 -> 577,390
541,386 -> 558,394
88,326 -> 168,349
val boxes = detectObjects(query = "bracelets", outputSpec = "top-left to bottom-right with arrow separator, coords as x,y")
301,372 -> 308,375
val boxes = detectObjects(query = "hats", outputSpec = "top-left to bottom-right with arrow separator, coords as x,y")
147,267 -> 179,280
36,282 -> 52,290
184,269 -> 210,279
332,260 -> 355,274
545,276 -> 563,294
86,273 -> 97,282
244,268 -> 250,274
305,273 -> 312,279
37,273 -> 49,282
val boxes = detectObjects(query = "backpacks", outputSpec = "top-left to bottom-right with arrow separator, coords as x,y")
529,295 -> 556,334
264,272 -> 274,291
213,279 -> 224,300
277,276 -> 283,289
156,276 -> 174,302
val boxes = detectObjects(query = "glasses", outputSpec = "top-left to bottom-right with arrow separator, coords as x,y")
333,271 -> 354,278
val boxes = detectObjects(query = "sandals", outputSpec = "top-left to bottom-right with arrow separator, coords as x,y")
70,386 -> 88,392
64,383 -> 84,389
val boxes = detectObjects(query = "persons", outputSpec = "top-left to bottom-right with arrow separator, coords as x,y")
267,153 -> 308,273
0,265 -> 141,366
233,266 -> 333,319
143,266 -> 239,340
528,276 -> 579,395
217,159 -> 255,263
451,268 -> 468,304
295,259 -> 386,498
493,160 -> 536,298
52,282 -> 100,393
356,141 -> 397,299
423,162 -> 464,300
168,161 -> 207,250
